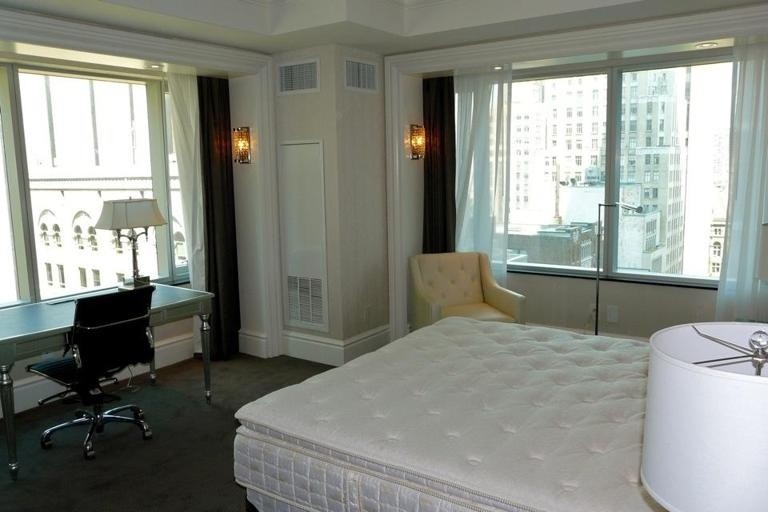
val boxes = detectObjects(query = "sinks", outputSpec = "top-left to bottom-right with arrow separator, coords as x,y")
410,252 -> 525,334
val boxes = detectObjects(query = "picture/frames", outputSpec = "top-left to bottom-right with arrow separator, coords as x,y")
1,284 -> 213,479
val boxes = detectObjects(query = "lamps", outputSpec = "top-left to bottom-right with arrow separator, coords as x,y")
25,286 -> 157,461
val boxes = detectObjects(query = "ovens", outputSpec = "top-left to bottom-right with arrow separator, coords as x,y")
234,315 -> 658,512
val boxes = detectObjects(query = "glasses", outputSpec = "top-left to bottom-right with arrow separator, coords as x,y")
409,122 -> 426,160
96,199 -> 169,291
757,223 -> 768,280
592,202 -> 643,337
640,321 -> 768,511
234,126 -> 251,168
